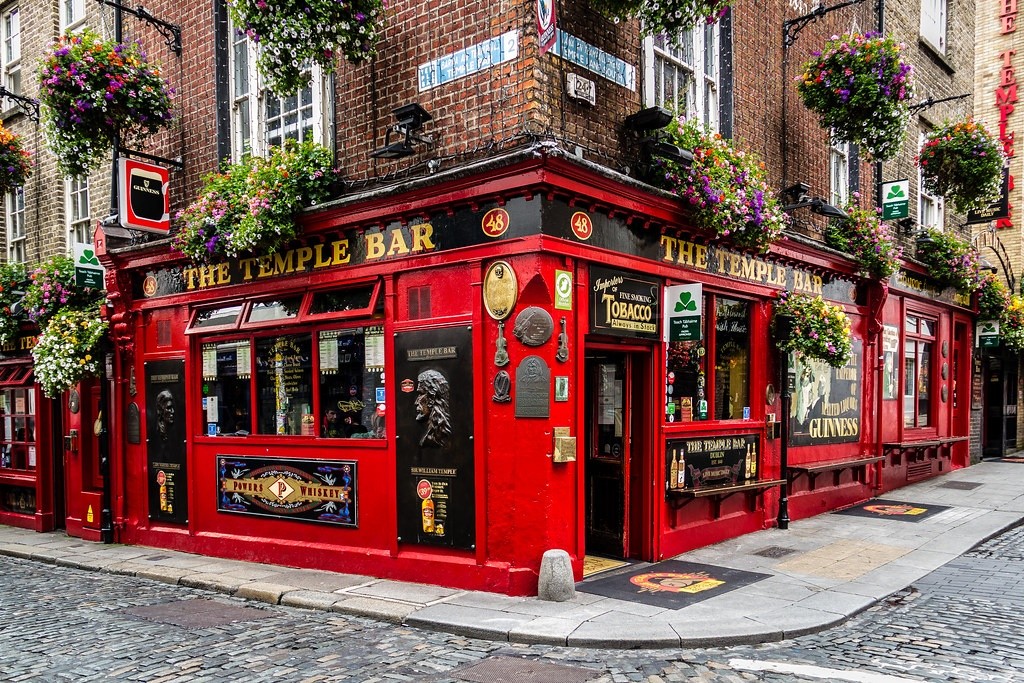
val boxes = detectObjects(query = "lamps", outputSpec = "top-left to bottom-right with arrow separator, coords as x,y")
897,217 -> 936,245
367,101 -> 433,160
626,104 -> 694,167
780,182 -> 850,221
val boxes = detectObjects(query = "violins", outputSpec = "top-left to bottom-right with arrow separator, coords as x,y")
556,317 -> 569,362
129,366 -> 137,397
494,320 -> 510,367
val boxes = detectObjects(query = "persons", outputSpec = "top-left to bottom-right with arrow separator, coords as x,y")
414,370 -> 453,452
320,407 -> 368,438
155,390 -> 174,440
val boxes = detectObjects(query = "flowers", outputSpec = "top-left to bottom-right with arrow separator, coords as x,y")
824,190 -> 903,278
767,285 -> 857,370
31,27 -> 176,183
913,115 -> 1011,216
223,0 -> 390,102
164,129 -> 341,267
0,256 -> 114,400
917,223 -> 1024,353
793,30 -> 915,164
587,0 -> 735,50
0,118 -> 37,197
650,91 -> 792,255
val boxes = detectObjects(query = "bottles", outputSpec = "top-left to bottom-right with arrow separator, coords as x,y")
751,443 -> 756,477
422,497 -> 434,532
670,449 -> 678,488
745,444 -> 751,478
678,449 -> 685,488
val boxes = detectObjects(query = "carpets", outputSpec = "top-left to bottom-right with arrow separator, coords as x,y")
984,456 -> 1024,463
831,498 -> 956,523
579,559 -> 776,611
582,555 -> 628,576
